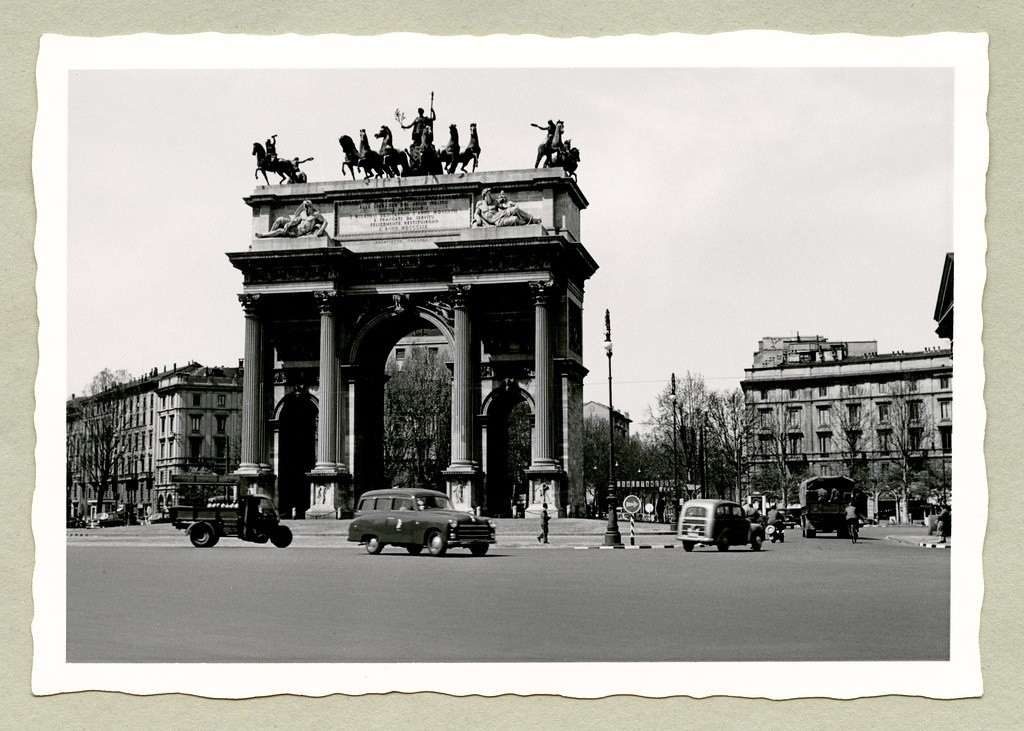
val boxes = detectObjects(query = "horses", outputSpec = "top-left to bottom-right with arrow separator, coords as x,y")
338,135 -> 360,180
252,142 -> 300,186
436,124 -> 460,174
374,125 -> 410,179
357,129 -> 383,180
547,147 -> 580,182
414,123 -> 436,175
458,123 -> 481,173
534,119 -> 572,169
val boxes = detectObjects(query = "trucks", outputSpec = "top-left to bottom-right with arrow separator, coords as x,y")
799,477 -> 857,539
167,494 -> 294,549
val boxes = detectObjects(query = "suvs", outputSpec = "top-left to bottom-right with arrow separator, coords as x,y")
347,486 -> 498,557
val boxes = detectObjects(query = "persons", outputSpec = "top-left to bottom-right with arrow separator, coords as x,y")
810,485 -> 827,503
936,504 -> 950,543
828,485 -> 841,503
254,199 -> 328,238
402,107 -> 437,145
290,156 -> 314,172
745,503 -> 761,518
265,134 -> 278,162
537,502 -> 551,543
531,120 -> 556,147
767,505 -> 785,529
474,188 -> 542,226
844,503 -> 859,535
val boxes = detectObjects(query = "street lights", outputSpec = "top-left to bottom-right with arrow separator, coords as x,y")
669,372 -> 679,530
931,427 -> 948,508
602,308 -> 624,550
700,407 -> 709,499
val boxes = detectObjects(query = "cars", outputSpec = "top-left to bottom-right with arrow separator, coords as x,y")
677,497 -> 764,554
778,511 -> 798,529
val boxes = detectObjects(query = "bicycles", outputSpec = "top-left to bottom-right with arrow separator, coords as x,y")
849,520 -> 861,544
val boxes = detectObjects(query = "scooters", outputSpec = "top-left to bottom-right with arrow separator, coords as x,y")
766,518 -> 789,543
